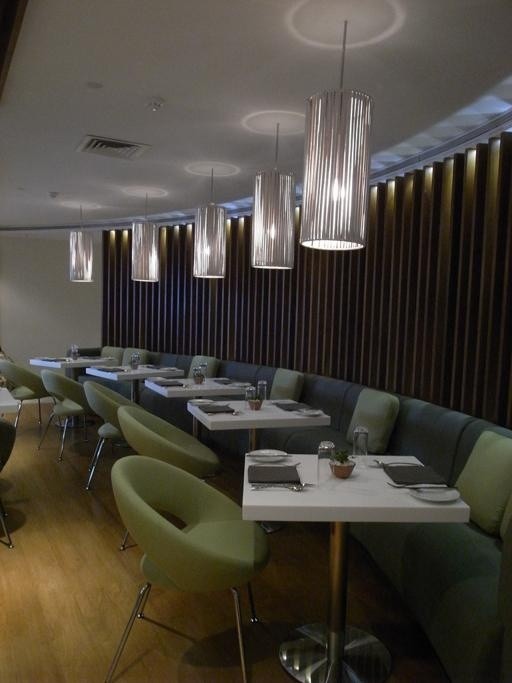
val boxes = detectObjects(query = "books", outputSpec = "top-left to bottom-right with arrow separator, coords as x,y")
198,403 -> 235,414
97,365 -> 126,372
271,401 -> 311,411
213,375 -> 238,387
44,358 -> 66,363
154,375 -> 185,389
248,464 -> 301,488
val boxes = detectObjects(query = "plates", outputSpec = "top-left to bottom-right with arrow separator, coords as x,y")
189,398 -> 214,405
249,448 -> 288,462
298,408 -> 323,417
408,483 -> 462,502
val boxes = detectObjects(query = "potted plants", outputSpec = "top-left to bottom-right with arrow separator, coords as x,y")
327,447 -> 357,479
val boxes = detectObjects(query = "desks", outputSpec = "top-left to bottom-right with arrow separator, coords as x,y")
0,385 -> 20,421
241,450 -> 471,683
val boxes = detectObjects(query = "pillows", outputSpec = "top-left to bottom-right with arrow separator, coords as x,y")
346,387 -> 399,455
451,425 -> 511,533
497,495 -> 511,549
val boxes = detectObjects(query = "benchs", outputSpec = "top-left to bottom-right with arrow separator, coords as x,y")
375,417 -> 512,682
287,384 -> 480,555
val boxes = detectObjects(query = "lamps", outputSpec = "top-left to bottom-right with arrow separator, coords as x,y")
249,122 -> 297,276
68,208 -> 95,283
190,167 -> 230,281
295,21 -> 373,256
131,190 -> 162,283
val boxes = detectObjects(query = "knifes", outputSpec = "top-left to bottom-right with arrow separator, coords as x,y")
247,453 -> 293,457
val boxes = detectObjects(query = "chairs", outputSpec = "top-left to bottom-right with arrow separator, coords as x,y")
107,454 -> 272,682
0,416 -> 19,549
35,371 -> 92,461
76,381 -> 139,490
0,360 -> 49,433
117,404 -> 222,552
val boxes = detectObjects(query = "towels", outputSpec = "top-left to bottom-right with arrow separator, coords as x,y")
249,464 -> 301,484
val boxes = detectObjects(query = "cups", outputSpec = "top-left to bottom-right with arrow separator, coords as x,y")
258,379 -> 267,407
244,385 -> 256,415
192,367 -> 201,389
316,440 -> 336,492
353,425 -> 370,471
198,361 -> 208,390
